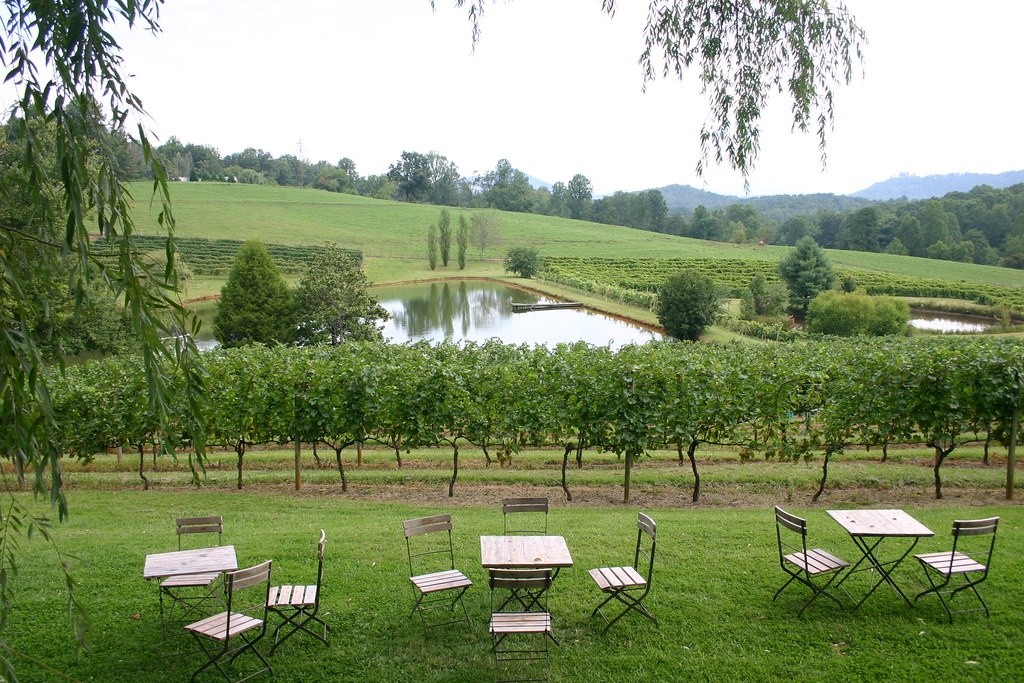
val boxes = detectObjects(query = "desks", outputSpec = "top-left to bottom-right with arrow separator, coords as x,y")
480,535 -> 572,620
143,545 -> 239,655
826,509 -> 935,611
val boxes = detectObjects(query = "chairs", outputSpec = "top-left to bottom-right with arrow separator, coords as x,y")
501,497 -> 549,535
587,512 -> 658,634
264,529 -> 335,658
159,516 -> 229,633
183,559 -> 274,683
773,506 -> 850,619
403,513 -> 473,638
913,516 -> 999,623
488,567 -> 559,683
